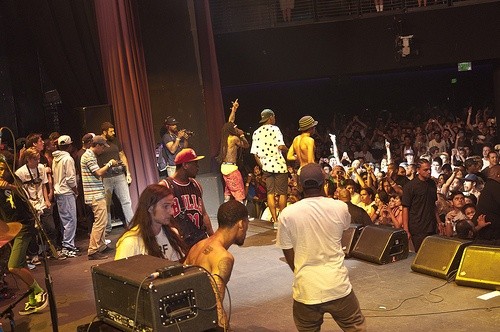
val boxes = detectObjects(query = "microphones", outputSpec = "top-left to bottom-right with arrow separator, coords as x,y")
243,132 -> 251,136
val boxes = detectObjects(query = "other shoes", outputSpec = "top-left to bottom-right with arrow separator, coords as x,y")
51,250 -> 67,259
18,289 -> 48,316
272,222 -> 278,229
26,257 -> 41,269
62,245 -> 80,258
105,231 -> 110,237
87,251 -> 109,259
103,247 -> 114,252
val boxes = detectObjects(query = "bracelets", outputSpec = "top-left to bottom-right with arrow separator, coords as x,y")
240,135 -> 244,137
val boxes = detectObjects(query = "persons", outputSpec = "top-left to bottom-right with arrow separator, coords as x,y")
0,122 -> 133,270
114,185 -> 187,265
159,114 -> 191,177
216,98 -> 255,221
250,109 -> 289,229
319,104 -> 500,253
287,116 -> 318,199
275,163 -> 368,332
159,148 -> 215,250
0,156 -> 48,315
243,165 -> 303,222
185,200 -> 249,327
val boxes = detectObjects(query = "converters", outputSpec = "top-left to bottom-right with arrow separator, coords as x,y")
157,263 -> 184,279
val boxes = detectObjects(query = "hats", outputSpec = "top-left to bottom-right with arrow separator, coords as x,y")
465,158 -> 477,169
260,109 -> 273,122
300,163 -> 323,188
298,116 -> 318,131
81,134 -> 93,143
57,135 -> 72,145
174,148 -> 205,164
92,136 -> 111,148
166,117 -> 179,125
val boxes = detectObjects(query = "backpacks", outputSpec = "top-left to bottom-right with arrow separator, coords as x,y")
154,133 -> 177,172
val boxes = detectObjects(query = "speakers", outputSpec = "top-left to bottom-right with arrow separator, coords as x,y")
352,225 -> 409,264
70,103 -> 116,145
411,235 -> 472,279
339,222 -> 363,256
455,243 -> 500,291
89,251 -> 218,332
